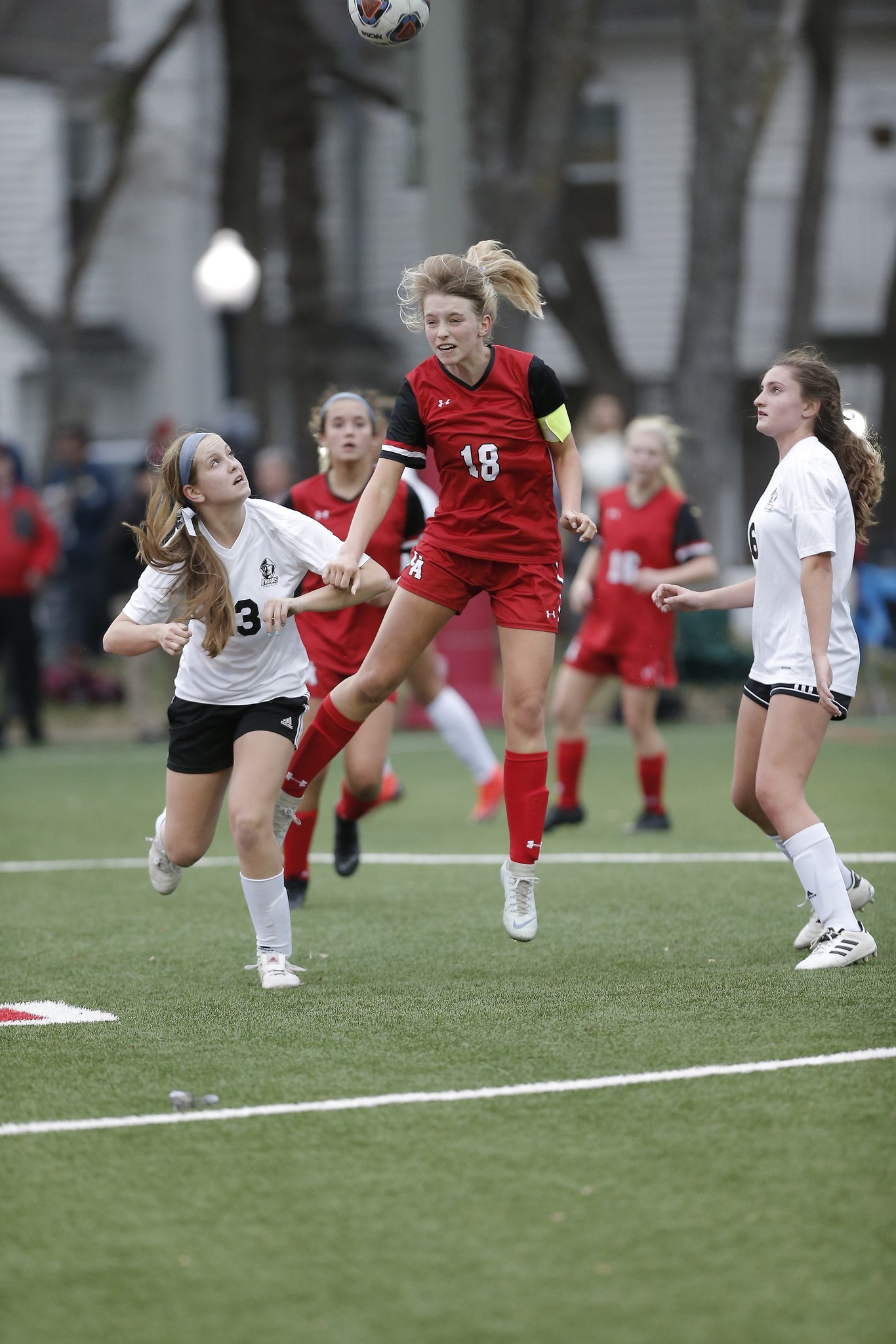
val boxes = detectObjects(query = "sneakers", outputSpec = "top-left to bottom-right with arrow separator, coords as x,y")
500,859 -> 539,942
794,921 -> 877,969
283,879 -> 304,908
797,871 -> 877,950
374,776 -> 400,804
471,766 -> 512,821
147,810 -> 183,895
272,805 -> 300,845
245,950 -> 306,990
334,815 -> 358,876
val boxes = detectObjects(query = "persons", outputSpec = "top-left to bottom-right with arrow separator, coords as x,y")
0,418 -> 309,749
373,394 -> 503,823
543,416 -> 719,832
270,238 -> 599,940
278,387 -> 426,910
650,346 -> 883,970
104,431 -> 391,989
552,380 -> 683,724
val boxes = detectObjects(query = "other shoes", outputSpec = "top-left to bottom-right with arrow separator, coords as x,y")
636,812 -> 665,833
541,806 -> 583,832
